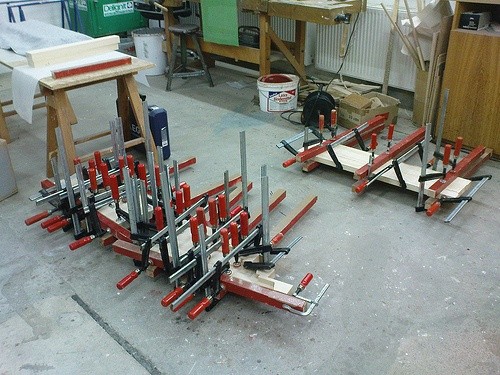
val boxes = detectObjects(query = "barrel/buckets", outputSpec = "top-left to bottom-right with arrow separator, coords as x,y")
257,74 -> 299,113
131,27 -> 168,75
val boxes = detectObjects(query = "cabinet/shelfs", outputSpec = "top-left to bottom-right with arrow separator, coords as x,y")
432,0 -> 500,162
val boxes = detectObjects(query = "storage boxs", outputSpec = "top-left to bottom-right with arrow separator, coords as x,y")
338,92 -> 400,129
412,68 -> 429,128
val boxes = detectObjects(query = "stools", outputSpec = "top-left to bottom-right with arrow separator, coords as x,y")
167,23 -> 214,90
